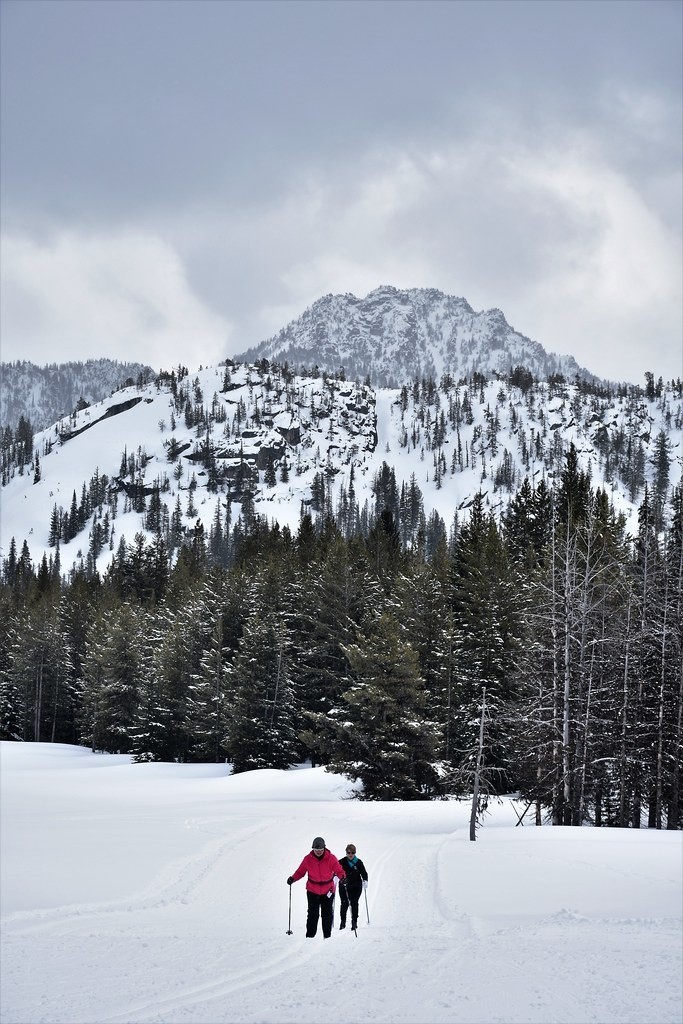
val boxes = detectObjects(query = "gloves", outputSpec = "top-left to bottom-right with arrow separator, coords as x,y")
287,877 -> 294,884
342,877 -> 347,884
363,880 -> 368,887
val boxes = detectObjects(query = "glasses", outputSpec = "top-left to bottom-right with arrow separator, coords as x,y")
347,851 -> 356,855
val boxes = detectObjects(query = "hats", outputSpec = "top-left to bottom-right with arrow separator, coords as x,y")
312,838 -> 325,849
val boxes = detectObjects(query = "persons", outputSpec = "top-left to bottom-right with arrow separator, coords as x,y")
287,837 -> 347,939
334,844 -> 368,931
